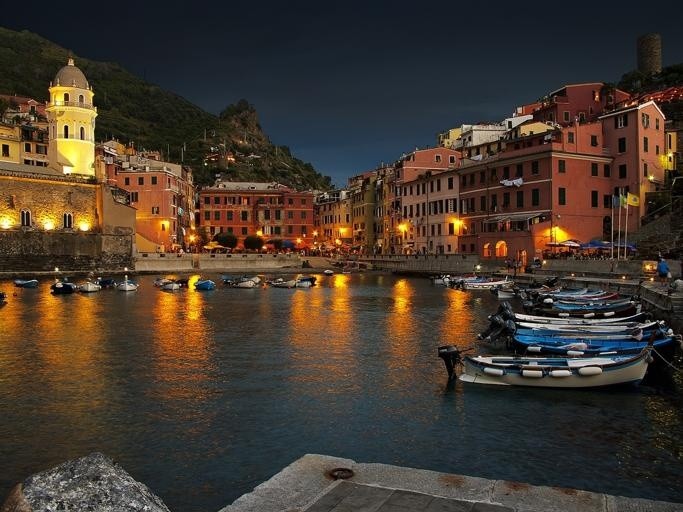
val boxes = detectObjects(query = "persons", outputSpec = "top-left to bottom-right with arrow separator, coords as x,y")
560,249 -> 613,262
511,259 -> 519,276
506,257 -> 512,274
656,257 -> 670,288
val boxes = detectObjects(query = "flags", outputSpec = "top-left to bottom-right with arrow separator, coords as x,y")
612,197 -> 618,207
617,193 -> 627,208
626,191 -> 639,207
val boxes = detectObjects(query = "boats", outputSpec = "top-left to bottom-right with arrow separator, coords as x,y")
14,279 -> 38,289
152,276 -> 190,291
324,269 -> 334,275
430,272 -> 674,341
49,281 -> 77,293
437,330 -> 656,390
94,279 -> 115,288
79,281 -> 102,292
264,276 -> 316,289
223,276 -> 262,288
113,279 -> 140,291
487,311 -> 673,356
194,279 -> 216,291
